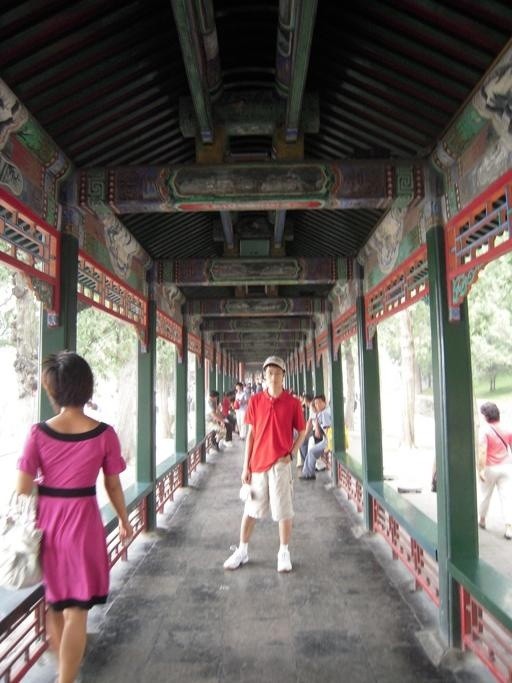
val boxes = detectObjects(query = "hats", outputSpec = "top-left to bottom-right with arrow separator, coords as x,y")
263,357 -> 287,372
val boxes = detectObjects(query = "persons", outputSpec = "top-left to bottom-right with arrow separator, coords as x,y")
204,380 -> 332,480
223,355 -> 308,573
475,400 -> 512,539
16,349 -> 134,682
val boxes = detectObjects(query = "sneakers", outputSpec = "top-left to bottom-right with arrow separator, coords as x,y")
210,437 -> 220,452
224,549 -> 249,570
299,474 -> 317,481
277,553 -> 294,573
